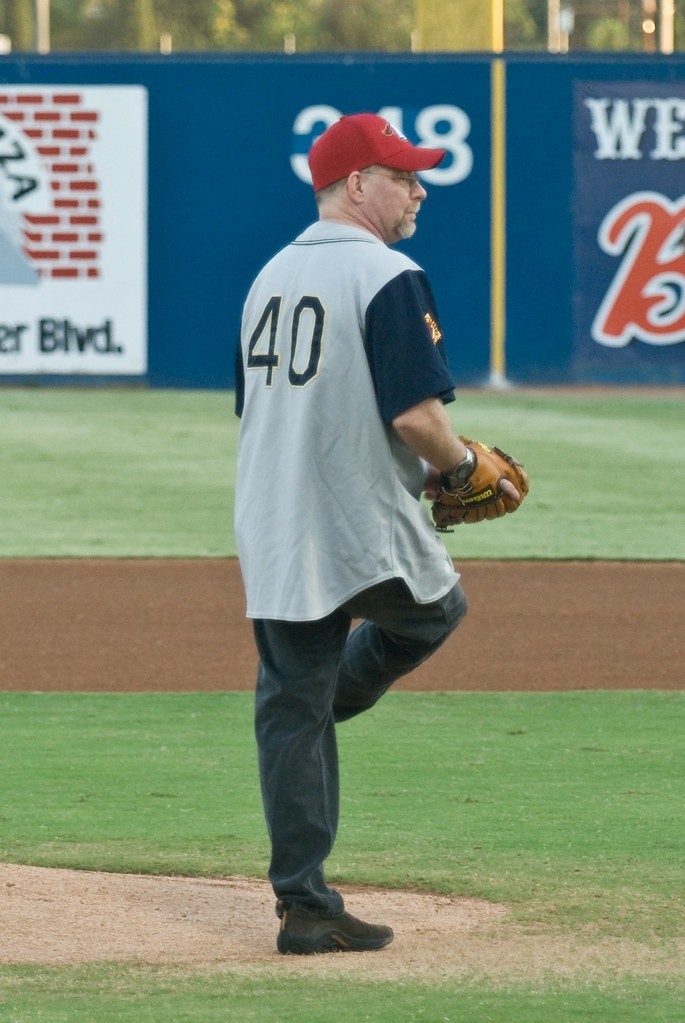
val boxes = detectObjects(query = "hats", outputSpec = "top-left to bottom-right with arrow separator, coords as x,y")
308,114 -> 446,192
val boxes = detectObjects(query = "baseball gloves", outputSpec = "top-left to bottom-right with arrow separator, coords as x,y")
432,434 -> 530,533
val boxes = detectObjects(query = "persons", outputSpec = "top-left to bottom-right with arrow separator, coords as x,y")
232,113 -> 529,956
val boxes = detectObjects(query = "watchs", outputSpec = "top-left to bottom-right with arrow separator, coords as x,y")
451,449 -> 475,481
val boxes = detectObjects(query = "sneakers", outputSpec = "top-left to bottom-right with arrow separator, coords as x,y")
276,909 -> 393,955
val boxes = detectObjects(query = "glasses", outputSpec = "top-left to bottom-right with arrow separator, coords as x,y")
346,170 -> 419,189
